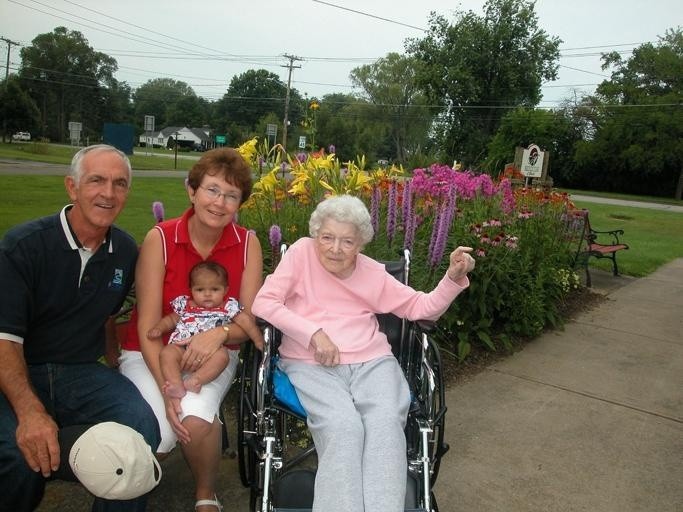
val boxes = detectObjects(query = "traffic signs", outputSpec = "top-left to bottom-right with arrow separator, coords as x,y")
216,136 -> 225,143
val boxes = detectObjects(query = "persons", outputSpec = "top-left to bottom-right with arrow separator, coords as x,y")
146,261 -> 263,398
120,145 -> 262,511
0,143 -> 162,511
252,193 -> 475,512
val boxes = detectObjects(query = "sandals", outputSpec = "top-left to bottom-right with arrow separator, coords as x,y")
194,492 -> 224,512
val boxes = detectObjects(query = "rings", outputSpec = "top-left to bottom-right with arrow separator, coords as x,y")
196,359 -> 202,362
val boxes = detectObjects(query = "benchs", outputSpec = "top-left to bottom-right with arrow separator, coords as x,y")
566,208 -> 630,288
113,304 -> 235,460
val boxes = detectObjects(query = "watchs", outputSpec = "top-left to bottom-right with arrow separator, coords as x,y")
216,325 -> 230,345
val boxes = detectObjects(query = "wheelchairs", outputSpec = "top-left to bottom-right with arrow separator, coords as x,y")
235,241 -> 453,512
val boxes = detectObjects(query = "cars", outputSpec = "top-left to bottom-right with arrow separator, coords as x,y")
11,131 -> 33,141
180,143 -> 206,152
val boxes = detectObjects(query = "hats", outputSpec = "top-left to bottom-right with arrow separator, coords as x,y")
55,421 -> 162,500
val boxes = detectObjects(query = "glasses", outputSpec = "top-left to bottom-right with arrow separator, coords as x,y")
200,184 -> 242,203
